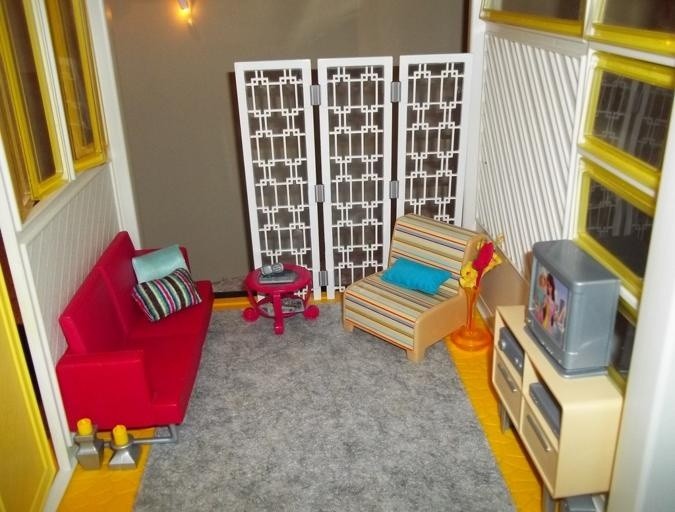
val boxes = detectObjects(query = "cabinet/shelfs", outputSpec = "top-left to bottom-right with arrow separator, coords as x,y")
487,306 -> 622,512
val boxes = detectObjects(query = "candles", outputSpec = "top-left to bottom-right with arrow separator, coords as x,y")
77,415 -> 130,446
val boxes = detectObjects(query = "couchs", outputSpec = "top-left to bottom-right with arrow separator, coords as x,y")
54,230 -> 216,443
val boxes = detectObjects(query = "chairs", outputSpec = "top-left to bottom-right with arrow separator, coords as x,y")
341,212 -> 490,362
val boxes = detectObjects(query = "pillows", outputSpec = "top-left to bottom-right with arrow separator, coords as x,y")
381,256 -> 452,296
131,244 -> 202,325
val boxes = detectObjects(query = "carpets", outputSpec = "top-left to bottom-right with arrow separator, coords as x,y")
133,303 -> 516,511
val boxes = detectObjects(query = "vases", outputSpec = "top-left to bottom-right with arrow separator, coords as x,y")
452,286 -> 492,351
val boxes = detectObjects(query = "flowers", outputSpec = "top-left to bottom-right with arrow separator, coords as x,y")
458,241 -> 495,329
